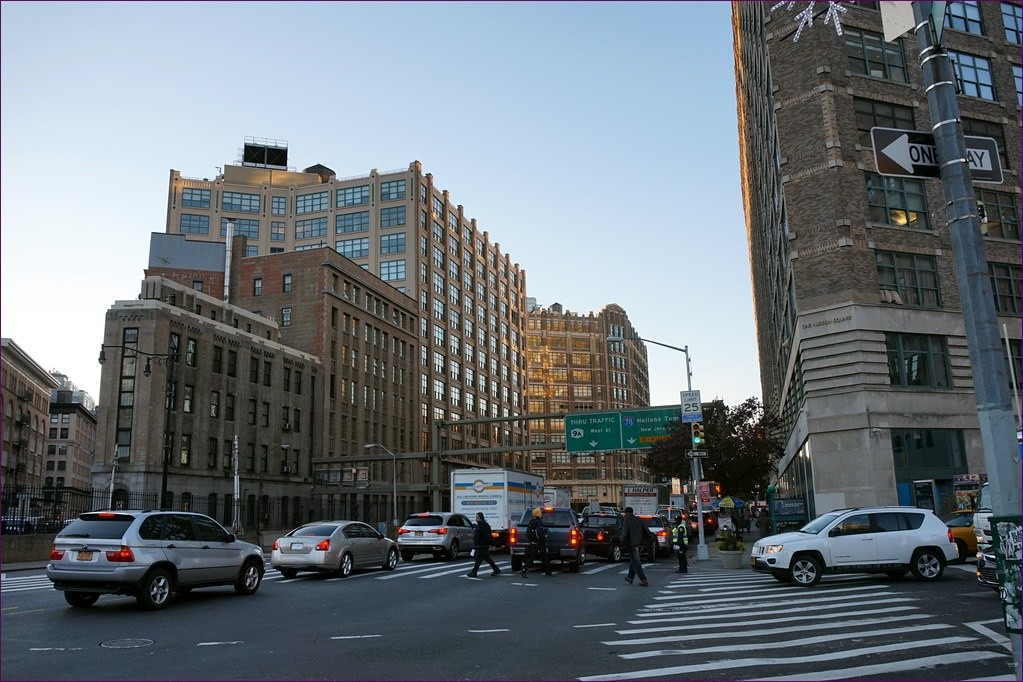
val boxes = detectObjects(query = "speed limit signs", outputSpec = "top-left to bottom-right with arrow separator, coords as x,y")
680,390 -> 702,414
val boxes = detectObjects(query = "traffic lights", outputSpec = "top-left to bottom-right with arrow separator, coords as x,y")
692,423 -> 706,446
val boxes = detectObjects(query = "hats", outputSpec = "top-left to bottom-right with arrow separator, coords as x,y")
674,515 -> 682,521
532,508 -> 542,518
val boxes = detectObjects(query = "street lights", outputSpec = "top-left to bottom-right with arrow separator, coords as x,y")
98,345 -> 183,512
363,443 -> 397,519
767,484 -> 777,534
606,335 -> 710,561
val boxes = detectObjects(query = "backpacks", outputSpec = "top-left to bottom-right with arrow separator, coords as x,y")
527,520 -> 540,540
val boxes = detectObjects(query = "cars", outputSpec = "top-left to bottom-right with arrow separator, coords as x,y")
1,515 -> 76,534
271,520 -> 399,578
656,504 -> 721,534
940,508 -> 979,565
396,512 -> 478,562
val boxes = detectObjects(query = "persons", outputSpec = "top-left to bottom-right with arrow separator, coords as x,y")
521,508 -> 554,577
776,502 -> 804,514
729,507 -> 773,537
468,512 -> 501,577
672,518 -> 688,573
621,507 -> 648,585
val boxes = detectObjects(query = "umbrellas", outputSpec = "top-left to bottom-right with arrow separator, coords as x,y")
711,497 -> 748,508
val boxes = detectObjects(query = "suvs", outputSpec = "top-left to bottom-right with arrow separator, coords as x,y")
635,515 -> 673,559
46,507 -> 267,612
575,506 -> 635,563
750,505 -> 962,587
508,506 -> 585,573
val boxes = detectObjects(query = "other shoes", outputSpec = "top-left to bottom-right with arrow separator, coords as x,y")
625,576 -> 633,584
491,570 -> 501,577
467,573 -> 476,577
639,579 -> 648,586
520,572 -> 528,578
675,570 -> 687,573
545,572 -> 554,577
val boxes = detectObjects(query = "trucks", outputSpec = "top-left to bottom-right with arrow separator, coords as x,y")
970,482 -> 1000,593
544,486 -> 572,509
450,466 -> 547,548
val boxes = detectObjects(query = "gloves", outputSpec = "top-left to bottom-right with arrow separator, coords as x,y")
675,545 -> 680,550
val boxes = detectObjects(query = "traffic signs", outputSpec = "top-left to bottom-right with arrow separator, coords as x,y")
870,126 -> 1004,185
620,404 -> 684,452
564,411 -> 622,454
685,449 -> 709,459
